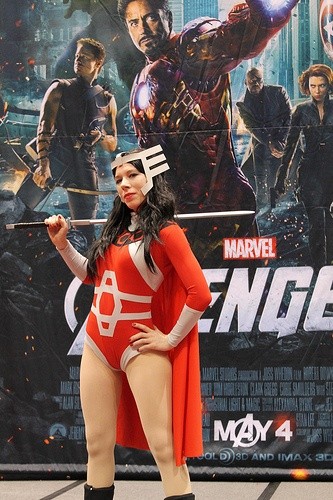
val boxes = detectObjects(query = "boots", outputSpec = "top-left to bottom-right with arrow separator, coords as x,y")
164,493 -> 195,500
84,483 -> 115,500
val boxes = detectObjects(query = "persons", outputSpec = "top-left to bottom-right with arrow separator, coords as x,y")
237,66 -> 292,202
45,145 -> 213,500
275,64 -> 333,272
118,0 -> 299,268
17,39 -> 117,254
